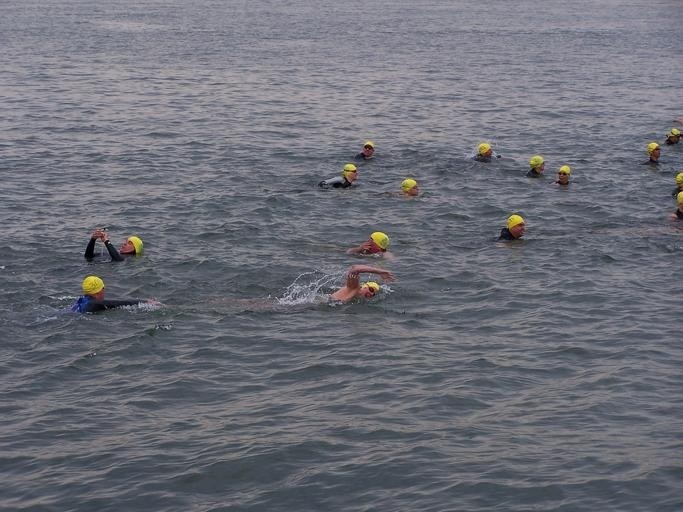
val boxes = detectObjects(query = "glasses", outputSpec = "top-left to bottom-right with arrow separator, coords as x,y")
366,283 -> 375,297
343,169 -> 357,174
363,146 -> 372,150
559,173 -> 567,175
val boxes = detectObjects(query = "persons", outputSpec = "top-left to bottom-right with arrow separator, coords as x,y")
550,165 -> 576,187
84,228 -> 145,263
67,275 -> 161,314
354,141 -> 379,162
329,265 -> 395,303
472,142 -> 494,163
525,155 -> 546,180
318,163 -> 359,190
643,126 -> 682,225
400,178 -> 420,198
347,232 -> 397,262
494,213 -> 527,242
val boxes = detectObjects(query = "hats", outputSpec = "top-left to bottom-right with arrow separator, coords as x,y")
508,214 -> 524,229
479,144 -> 490,154
676,173 -> 683,183
402,179 -> 417,192
531,156 -> 543,169
128,236 -> 144,253
671,129 -> 681,136
677,192 -> 683,203
363,282 -> 379,293
559,166 -> 570,174
344,164 -> 356,176
370,232 -> 389,249
648,143 -> 659,154
364,142 -> 374,150
83,276 -> 104,295
